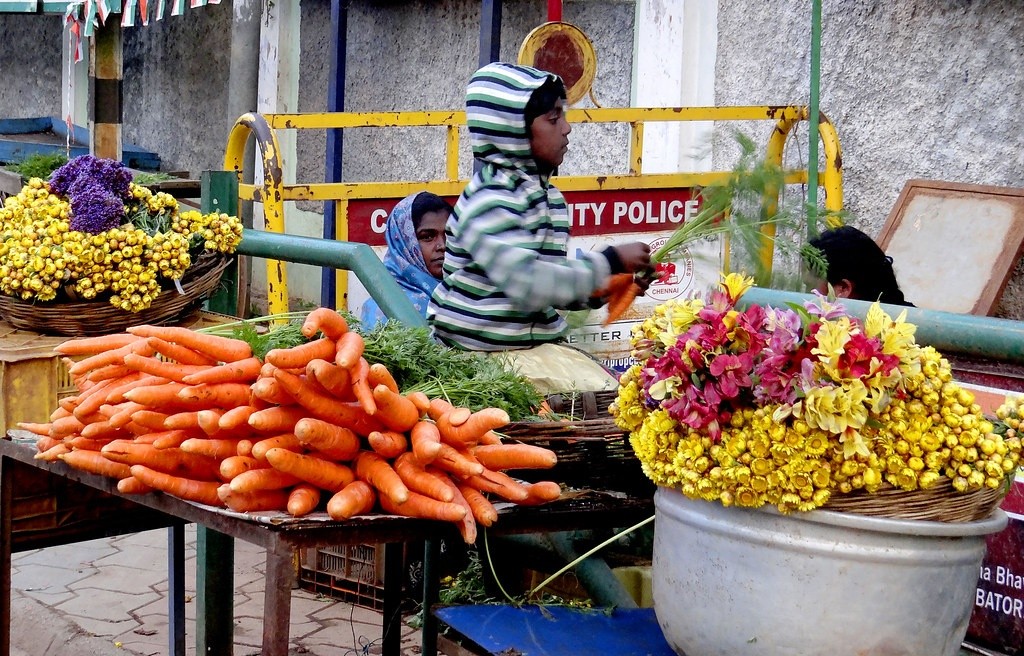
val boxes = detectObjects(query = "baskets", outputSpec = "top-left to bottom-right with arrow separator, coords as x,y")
491,391 -> 639,463
0,248 -> 234,337
816,414 -> 1017,523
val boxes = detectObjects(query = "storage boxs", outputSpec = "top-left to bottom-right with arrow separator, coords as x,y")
292,539 -> 385,613
1,318 -> 255,438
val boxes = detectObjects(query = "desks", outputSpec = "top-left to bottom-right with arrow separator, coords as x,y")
0,435 -> 652,656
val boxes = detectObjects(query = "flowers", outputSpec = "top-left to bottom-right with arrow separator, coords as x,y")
0,155 -> 246,314
607,270 -> 1023,513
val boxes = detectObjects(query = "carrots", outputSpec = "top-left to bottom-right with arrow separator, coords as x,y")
589,273 -> 641,328
15,307 -> 569,544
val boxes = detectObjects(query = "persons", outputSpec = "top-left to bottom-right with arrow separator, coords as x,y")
357,192 -> 454,327
798,225 -> 915,307
425,62 -> 650,389
